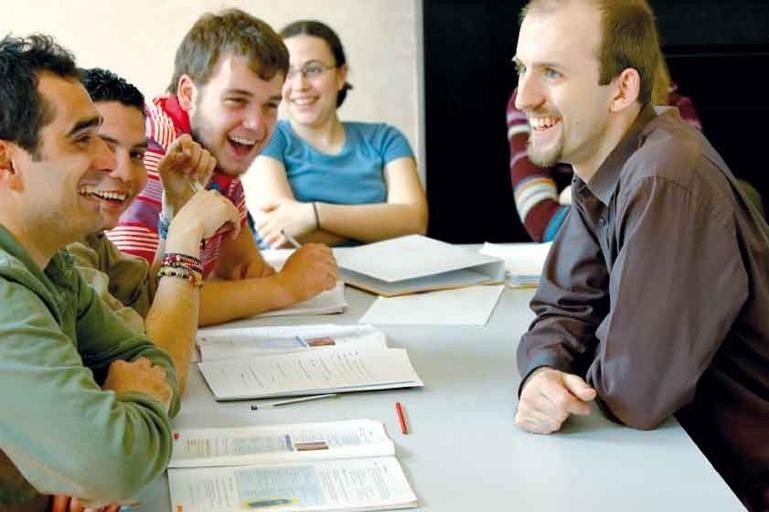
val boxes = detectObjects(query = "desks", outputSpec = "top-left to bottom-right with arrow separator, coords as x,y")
80,240 -> 749,512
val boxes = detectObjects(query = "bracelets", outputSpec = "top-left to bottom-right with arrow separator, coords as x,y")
312,202 -> 320,230
156,252 -> 207,288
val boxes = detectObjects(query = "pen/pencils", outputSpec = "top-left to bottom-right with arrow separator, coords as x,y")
182,171 -> 204,193
250,393 -> 341,410
396,401 -> 407,433
281,230 -> 303,250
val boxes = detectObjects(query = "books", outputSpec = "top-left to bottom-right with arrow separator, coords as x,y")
477,242 -> 554,289
165,419 -> 417,511
249,274 -> 348,318
192,320 -> 422,407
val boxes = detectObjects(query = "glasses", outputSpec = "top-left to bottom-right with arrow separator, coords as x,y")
286,59 -> 341,81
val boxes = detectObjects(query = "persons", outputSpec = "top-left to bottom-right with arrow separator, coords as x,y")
506,76 -> 702,242
0,33 -> 180,512
514,0 -> 769,512
103,7 -> 340,327
240,20 -> 429,255
66,68 -> 239,421
